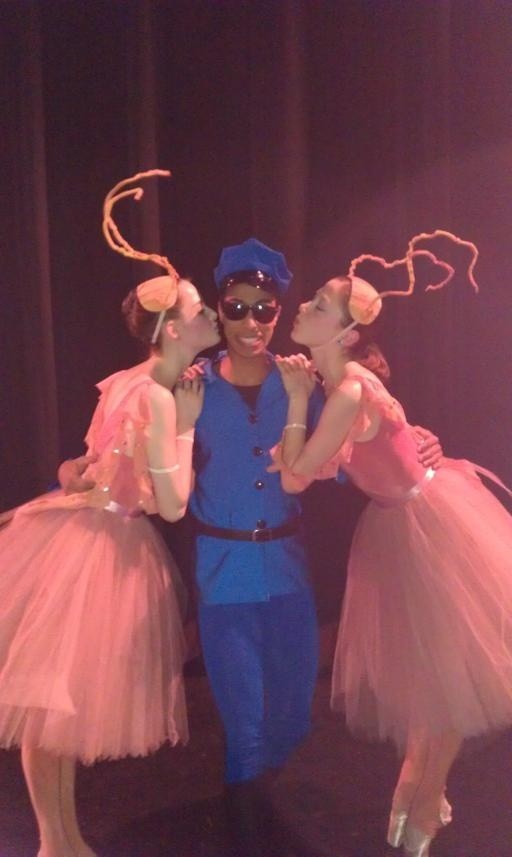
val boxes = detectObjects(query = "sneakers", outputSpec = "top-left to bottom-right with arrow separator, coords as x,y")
388,791 -> 454,857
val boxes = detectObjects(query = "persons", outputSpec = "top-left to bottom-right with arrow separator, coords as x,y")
55,235 -> 447,855
0,274 -> 223,856
264,274 -> 511,856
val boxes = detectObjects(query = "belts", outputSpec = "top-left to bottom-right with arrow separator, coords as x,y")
191,514 -> 303,543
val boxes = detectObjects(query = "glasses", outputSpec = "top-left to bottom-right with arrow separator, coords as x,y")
219,298 -> 282,324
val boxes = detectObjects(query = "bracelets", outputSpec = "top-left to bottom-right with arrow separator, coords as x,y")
284,422 -> 308,430
178,435 -> 194,441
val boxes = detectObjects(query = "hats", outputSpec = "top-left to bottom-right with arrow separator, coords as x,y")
212,238 -> 292,299
